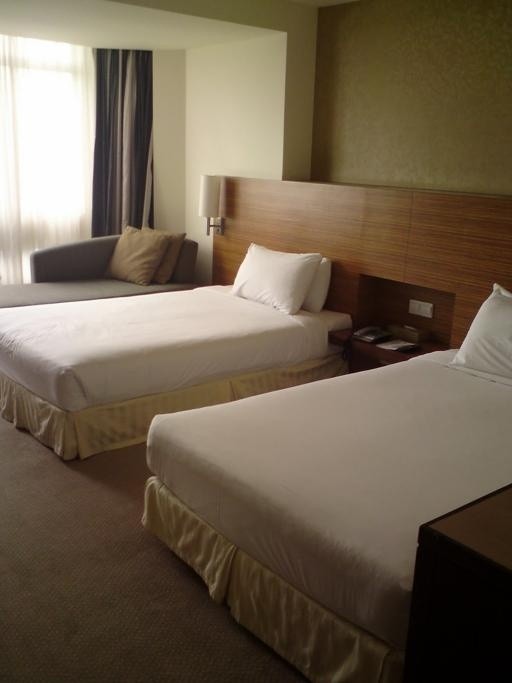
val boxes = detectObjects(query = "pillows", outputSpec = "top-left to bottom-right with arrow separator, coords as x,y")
232,243 -> 322,316
103,225 -> 173,287
445,282 -> 512,387
302,257 -> 331,314
141,226 -> 187,284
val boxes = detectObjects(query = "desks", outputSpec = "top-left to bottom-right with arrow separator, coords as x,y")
406,482 -> 512,682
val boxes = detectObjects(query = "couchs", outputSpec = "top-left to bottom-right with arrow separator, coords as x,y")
1,235 -> 198,307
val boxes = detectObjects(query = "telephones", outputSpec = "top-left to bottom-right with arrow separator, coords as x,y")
350,325 -> 393,345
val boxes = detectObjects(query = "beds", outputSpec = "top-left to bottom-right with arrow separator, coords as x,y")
0,285 -> 353,461
141,348 -> 511,683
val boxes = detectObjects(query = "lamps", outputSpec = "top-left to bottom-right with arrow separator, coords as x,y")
198,174 -> 225,236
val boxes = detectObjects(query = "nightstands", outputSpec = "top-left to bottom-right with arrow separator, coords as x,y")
328,326 -> 447,373
